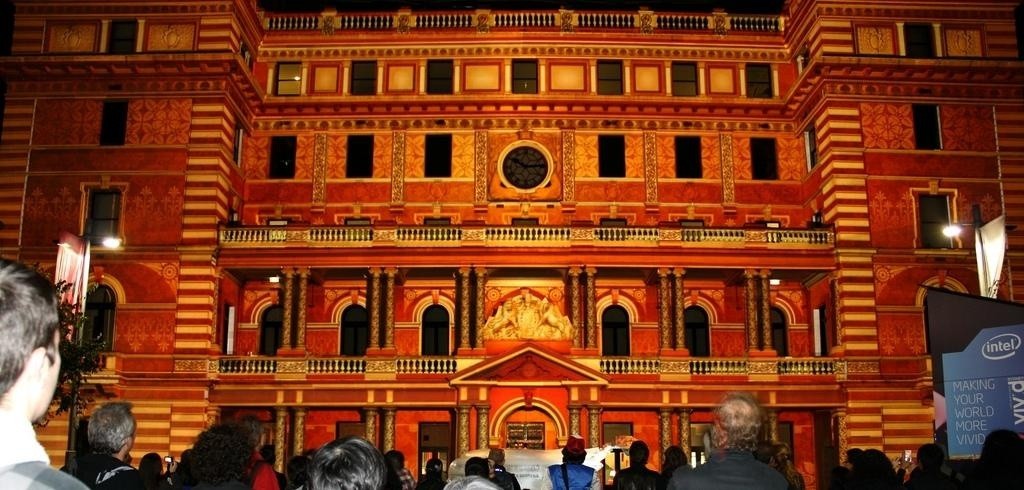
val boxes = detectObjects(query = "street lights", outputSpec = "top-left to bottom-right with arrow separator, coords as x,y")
51,218 -> 124,479
942,202 -> 1017,300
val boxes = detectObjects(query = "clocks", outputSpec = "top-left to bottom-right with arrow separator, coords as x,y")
497,140 -> 554,194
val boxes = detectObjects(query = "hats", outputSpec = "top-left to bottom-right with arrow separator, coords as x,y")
566,434 -> 585,456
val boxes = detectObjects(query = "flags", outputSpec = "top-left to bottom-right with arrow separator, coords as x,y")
918,283 -> 1023,460
972,213 -> 1007,300
51,231 -> 90,346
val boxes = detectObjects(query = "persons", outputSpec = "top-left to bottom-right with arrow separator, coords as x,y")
57,418 -> 94,478
125,389 -> 1024,490
0,255 -> 91,490
76,400 -> 143,489
